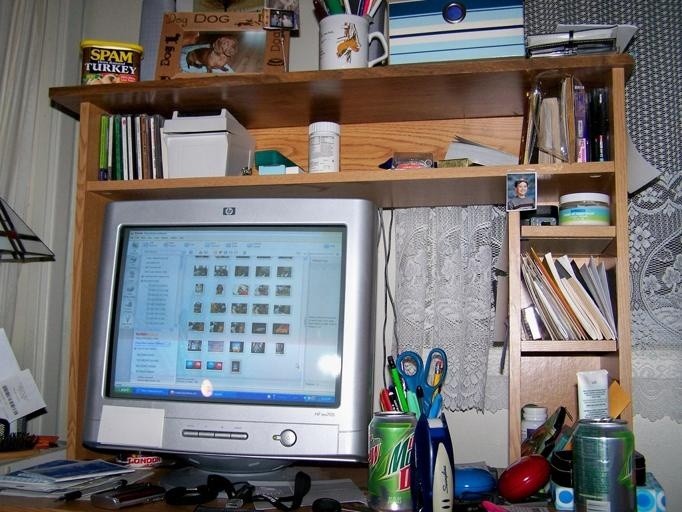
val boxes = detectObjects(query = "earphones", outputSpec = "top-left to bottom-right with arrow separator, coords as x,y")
312,497 -> 342,512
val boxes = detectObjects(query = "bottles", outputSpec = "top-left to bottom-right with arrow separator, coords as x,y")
520,405 -> 547,446
559,192 -> 612,225
307,119 -> 342,175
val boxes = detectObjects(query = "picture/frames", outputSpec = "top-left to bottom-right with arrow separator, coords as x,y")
154,12 -> 289,80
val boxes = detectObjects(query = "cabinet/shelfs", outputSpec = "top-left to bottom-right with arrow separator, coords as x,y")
0,53 -> 635,512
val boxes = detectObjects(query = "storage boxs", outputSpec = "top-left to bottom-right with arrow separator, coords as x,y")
387,0 -> 526,66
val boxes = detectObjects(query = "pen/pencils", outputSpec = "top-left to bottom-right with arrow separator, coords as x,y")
341,508 -> 361,512
53,479 -> 128,503
380,355 -> 443,418
311,0 -> 383,22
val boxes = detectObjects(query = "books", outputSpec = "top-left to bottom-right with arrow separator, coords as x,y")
99,112 -> 165,180
524,77 -> 611,165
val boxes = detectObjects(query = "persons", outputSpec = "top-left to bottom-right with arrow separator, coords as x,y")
269,11 -> 293,29
506,178 -> 535,211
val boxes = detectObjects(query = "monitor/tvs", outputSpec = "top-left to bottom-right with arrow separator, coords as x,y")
81,198 -> 380,496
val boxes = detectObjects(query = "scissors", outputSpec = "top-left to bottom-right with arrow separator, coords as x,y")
395,348 -> 447,419
343,502 -> 375,512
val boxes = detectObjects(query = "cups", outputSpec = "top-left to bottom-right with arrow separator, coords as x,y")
318,13 -> 389,71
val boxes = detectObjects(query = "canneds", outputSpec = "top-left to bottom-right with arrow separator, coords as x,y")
572,417 -> 636,512
367,411 -> 417,512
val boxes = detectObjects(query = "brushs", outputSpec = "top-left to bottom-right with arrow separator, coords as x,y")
0,431 -> 40,458
